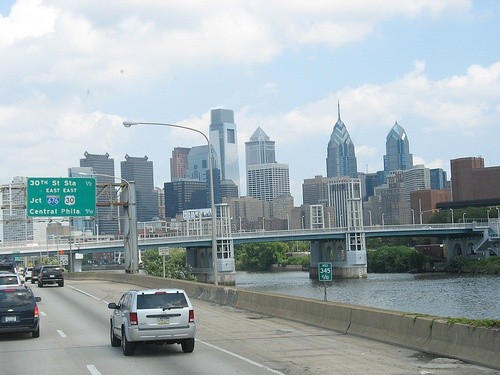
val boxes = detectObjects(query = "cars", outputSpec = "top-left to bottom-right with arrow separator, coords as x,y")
0,264 -> 65,288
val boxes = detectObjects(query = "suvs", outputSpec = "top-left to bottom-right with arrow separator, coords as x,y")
107,288 -> 197,357
0,284 -> 41,339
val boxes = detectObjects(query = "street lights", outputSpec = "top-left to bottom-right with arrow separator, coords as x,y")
78,172 -> 132,272
411,209 -> 414,229
301,216 -> 305,234
340,214 -> 343,232
463,213 -> 466,228
238,216 -> 241,231
262,217 -> 266,236
450,208 -> 453,226
286,214 -> 289,231
382,214 -> 385,230
369,210 -> 372,230
419,212 -> 423,225
123,120 -> 218,286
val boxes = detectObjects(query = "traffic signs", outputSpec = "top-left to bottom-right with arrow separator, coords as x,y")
26,177 -> 96,216
